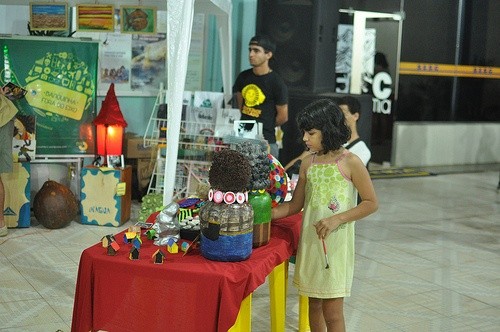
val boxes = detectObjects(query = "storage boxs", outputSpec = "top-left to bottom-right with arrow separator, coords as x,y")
126,138 -> 160,188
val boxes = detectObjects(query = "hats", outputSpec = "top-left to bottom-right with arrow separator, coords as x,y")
249,34 -> 276,53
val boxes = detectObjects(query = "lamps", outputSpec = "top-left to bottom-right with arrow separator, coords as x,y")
92,82 -> 128,166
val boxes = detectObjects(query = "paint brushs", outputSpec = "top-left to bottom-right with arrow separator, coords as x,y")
322,235 -> 329,268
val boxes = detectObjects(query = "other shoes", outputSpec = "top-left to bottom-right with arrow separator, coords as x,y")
0,226 -> 8,236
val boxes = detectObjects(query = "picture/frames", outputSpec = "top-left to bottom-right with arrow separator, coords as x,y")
120,5 -> 157,34
76,3 -> 115,32
29,2 -> 68,30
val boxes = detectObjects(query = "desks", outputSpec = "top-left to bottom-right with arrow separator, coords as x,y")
79,164 -> 132,227
71,209 -> 310,332
0,161 -> 32,228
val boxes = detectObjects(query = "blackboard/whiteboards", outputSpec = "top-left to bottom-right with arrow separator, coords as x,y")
0,35 -> 101,157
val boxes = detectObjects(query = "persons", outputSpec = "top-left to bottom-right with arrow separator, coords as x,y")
373,53 -> 388,144
0,94 -> 19,236
284,97 -> 371,172
271,98 -> 378,332
233,33 -> 289,159
